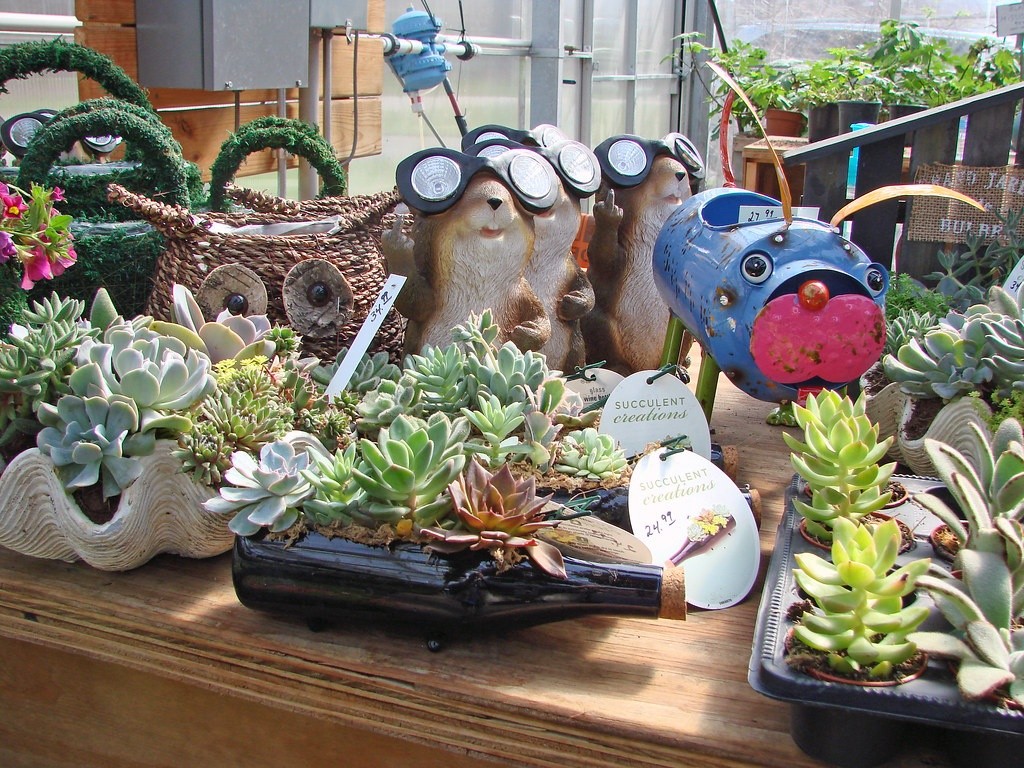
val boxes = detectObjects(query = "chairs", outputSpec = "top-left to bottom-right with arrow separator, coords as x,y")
780,79 -> 1024,289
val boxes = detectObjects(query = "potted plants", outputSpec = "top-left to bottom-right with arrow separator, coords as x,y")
0,8 -> 1024,768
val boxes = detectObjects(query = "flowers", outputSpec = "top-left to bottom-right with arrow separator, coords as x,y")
0,177 -> 80,289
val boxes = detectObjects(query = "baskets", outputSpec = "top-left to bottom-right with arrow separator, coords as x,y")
103,119 -> 416,366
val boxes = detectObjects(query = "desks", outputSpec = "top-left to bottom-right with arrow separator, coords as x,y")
742,137 -> 912,207
